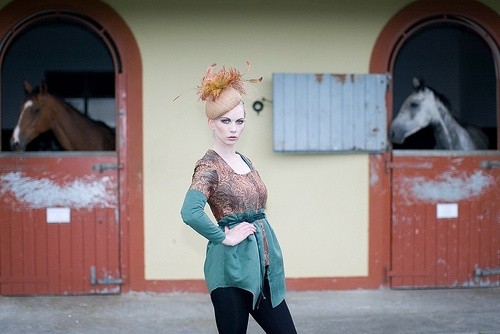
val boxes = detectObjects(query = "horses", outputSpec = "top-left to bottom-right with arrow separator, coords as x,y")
9,80 -> 116,153
387,77 -> 495,151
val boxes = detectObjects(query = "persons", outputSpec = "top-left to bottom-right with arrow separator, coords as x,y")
181,60 -> 297,334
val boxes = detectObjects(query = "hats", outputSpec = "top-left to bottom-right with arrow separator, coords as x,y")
195,62 -> 246,120
